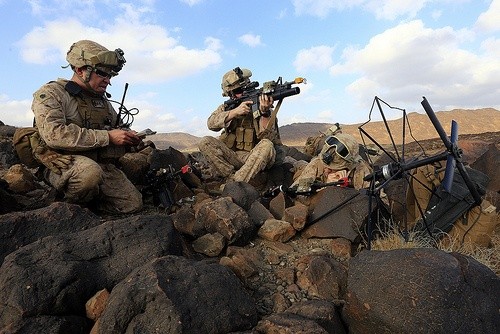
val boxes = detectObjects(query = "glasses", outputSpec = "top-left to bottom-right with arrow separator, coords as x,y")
92,67 -> 112,79
228,88 -> 243,96
324,136 -> 352,161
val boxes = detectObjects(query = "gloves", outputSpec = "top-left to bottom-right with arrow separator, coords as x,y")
35,147 -> 72,175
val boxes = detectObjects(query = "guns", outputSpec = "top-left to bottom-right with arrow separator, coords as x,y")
265,177 -> 353,198
141,164 -> 193,214
223,77 -> 306,120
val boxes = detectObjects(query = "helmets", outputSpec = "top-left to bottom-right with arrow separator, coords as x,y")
221,70 -> 251,97
66,40 -> 118,77
319,133 -> 360,171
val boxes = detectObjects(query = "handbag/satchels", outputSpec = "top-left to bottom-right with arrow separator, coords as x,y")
13,128 -> 44,169
218,131 -> 237,153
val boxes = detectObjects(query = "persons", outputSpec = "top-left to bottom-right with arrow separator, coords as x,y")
287,133 -> 384,199
196,66 -> 285,196
13,40 -> 146,215
